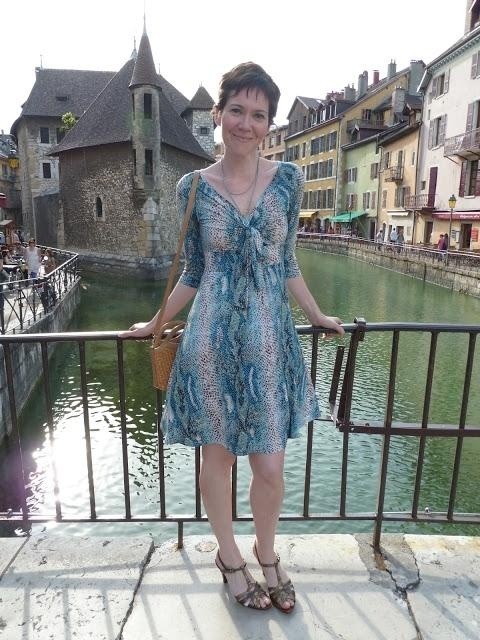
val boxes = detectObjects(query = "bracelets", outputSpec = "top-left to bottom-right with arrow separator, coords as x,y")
219,154 -> 261,218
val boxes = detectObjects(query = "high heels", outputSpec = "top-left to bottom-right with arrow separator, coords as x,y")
215,549 -> 272,611
254,539 -> 295,613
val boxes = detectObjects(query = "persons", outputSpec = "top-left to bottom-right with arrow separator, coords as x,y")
436,234 -> 445,250
397,230 -> 405,253
1,229 -> 56,278
442,233 -> 449,250
375,229 -> 384,251
118,61 -> 345,612
389,228 -> 397,247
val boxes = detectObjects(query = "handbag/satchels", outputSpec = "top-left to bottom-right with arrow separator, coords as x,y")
150,320 -> 186,391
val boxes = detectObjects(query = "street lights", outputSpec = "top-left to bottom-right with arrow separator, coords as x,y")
8,149 -> 20,232
444,194 -> 457,250
